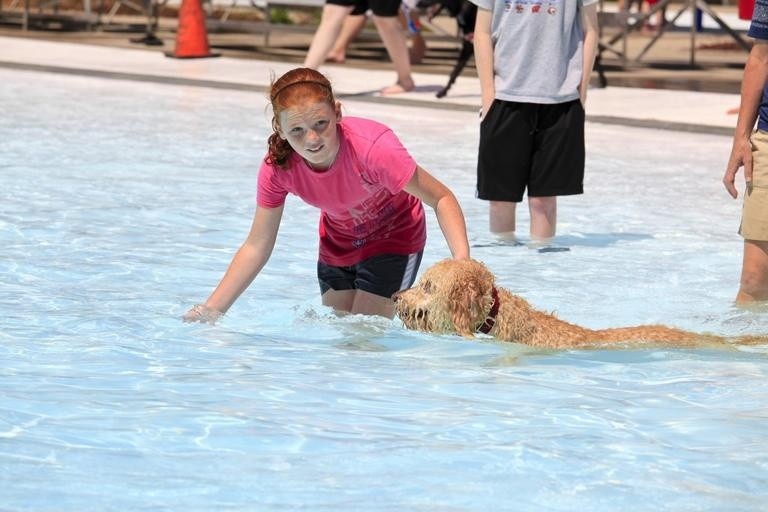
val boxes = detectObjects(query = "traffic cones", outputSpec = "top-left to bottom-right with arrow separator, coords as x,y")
161,0 -> 224,59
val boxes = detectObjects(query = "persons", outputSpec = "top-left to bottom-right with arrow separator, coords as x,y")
722,1 -> 768,302
325,0 -> 371,63
178,66 -> 473,324
465,0 -> 599,238
303,0 -> 414,96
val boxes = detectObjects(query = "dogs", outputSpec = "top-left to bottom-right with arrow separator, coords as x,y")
387,256 -> 768,369
